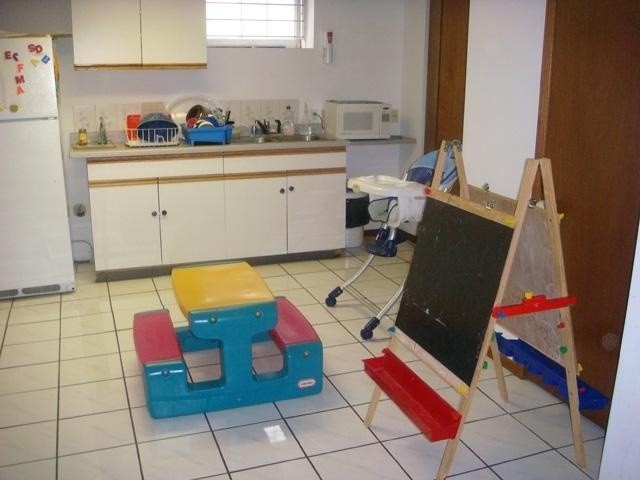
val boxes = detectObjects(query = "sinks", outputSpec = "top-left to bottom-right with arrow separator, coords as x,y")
232,133 -> 281,143
272,133 -> 336,141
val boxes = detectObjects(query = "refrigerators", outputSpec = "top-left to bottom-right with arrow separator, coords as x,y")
1,36 -> 77,301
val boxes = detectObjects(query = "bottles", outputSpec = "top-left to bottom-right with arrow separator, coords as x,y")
98,118 -> 107,143
78,129 -> 87,145
282,105 -> 295,136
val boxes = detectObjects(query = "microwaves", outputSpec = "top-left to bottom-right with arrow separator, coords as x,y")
324,100 -> 390,139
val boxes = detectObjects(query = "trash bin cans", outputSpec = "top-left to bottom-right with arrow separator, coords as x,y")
346,188 -> 369,248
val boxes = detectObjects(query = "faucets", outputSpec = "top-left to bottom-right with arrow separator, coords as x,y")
275,119 -> 281,133
254,119 -> 271,133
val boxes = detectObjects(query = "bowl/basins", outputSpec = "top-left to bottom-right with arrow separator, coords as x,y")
187,117 -> 215,128
186,105 -> 213,120
136,114 -> 179,140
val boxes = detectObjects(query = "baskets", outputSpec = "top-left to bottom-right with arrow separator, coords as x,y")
123,111 -> 181,147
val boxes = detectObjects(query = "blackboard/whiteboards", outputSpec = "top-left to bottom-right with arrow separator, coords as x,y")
387,186 -> 518,398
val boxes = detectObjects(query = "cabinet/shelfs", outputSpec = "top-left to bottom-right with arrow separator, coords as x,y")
70,1 -> 208,71
223,145 -> 347,266
86,153 -> 222,283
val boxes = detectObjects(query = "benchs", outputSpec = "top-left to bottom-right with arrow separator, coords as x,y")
269,295 -> 323,402
132,309 -> 190,420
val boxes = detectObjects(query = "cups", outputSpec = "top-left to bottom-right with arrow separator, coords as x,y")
127,115 -> 139,139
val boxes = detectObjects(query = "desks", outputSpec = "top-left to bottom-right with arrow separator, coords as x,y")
169,261 -> 278,413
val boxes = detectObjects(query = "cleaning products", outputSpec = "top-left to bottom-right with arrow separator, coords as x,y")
98,117 -> 107,143
78,112 -> 87,144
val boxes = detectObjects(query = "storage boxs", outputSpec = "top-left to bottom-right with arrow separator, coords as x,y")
180,122 -> 232,146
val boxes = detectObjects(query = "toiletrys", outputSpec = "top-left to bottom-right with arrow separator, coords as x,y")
281,106 -> 297,137
297,102 -> 313,135
